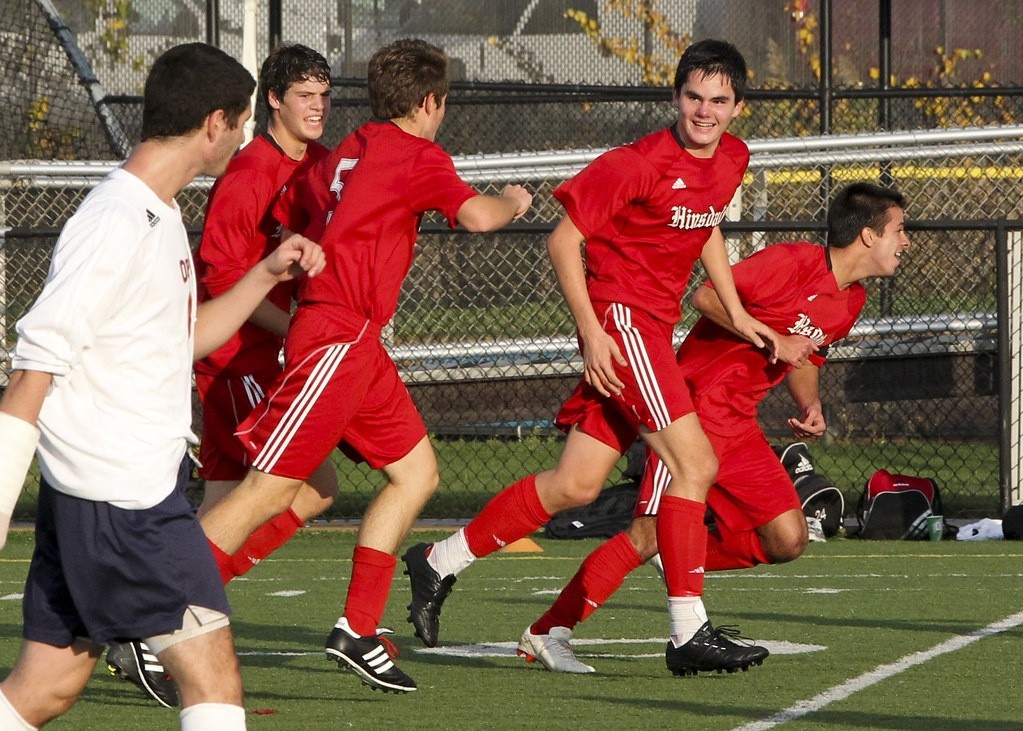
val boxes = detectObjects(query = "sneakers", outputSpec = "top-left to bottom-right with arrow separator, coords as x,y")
106,639 -> 178,708
517,623 -> 597,673
665,619 -> 769,677
325,614 -> 418,694
401,543 -> 456,647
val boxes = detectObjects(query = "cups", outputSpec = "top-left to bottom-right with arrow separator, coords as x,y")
927,516 -> 943,541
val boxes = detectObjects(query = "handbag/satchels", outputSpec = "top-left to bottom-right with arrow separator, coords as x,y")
774,442 -> 846,541
544,482 -> 638,537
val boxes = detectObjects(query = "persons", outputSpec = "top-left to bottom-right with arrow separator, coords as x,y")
522,182 -> 907,674
193,44 -> 339,584
104,40 -> 527,712
0,42 -> 327,731
396,38 -> 781,671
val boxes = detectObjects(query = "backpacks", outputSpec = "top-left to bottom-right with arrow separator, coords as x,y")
856,469 -> 946,544
